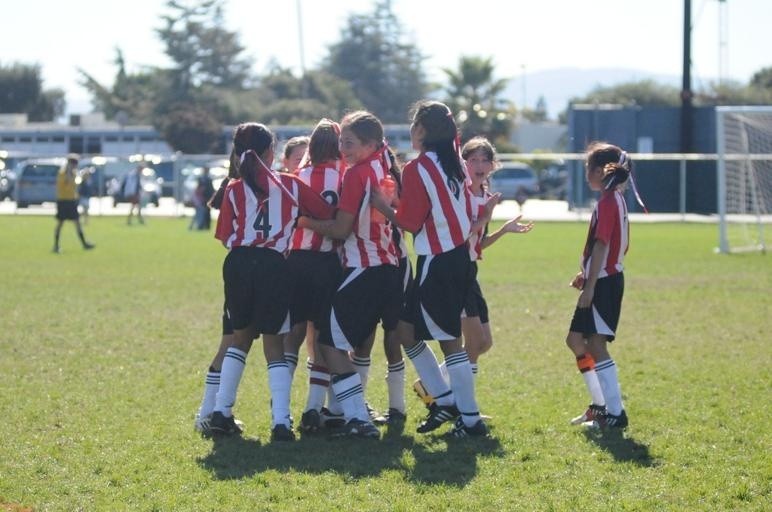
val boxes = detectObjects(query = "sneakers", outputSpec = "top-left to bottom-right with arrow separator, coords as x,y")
195,412 -> 244,437
444,417 -> 486,441
414,378 -> 460,435
271,415 -> 296,441
580,409 -> 628,432
571,406 -> 605,426
297,402 -> 407,441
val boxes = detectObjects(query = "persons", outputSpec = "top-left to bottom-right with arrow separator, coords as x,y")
413,136 -> 533,425
368,101 -> 490,442
77,170 -> 93,217
190,110 -> 413,440
50,155 -> 95,254
127,166 -> 147,225
564,145 -> 639,433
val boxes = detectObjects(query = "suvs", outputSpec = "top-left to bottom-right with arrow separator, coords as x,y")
539,159 -> 570,200
486,162 -> 542,208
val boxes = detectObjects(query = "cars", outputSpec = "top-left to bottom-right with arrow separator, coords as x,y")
0,151 -> 227,214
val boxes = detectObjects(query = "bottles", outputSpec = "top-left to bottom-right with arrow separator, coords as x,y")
371,175 -> 394,225
